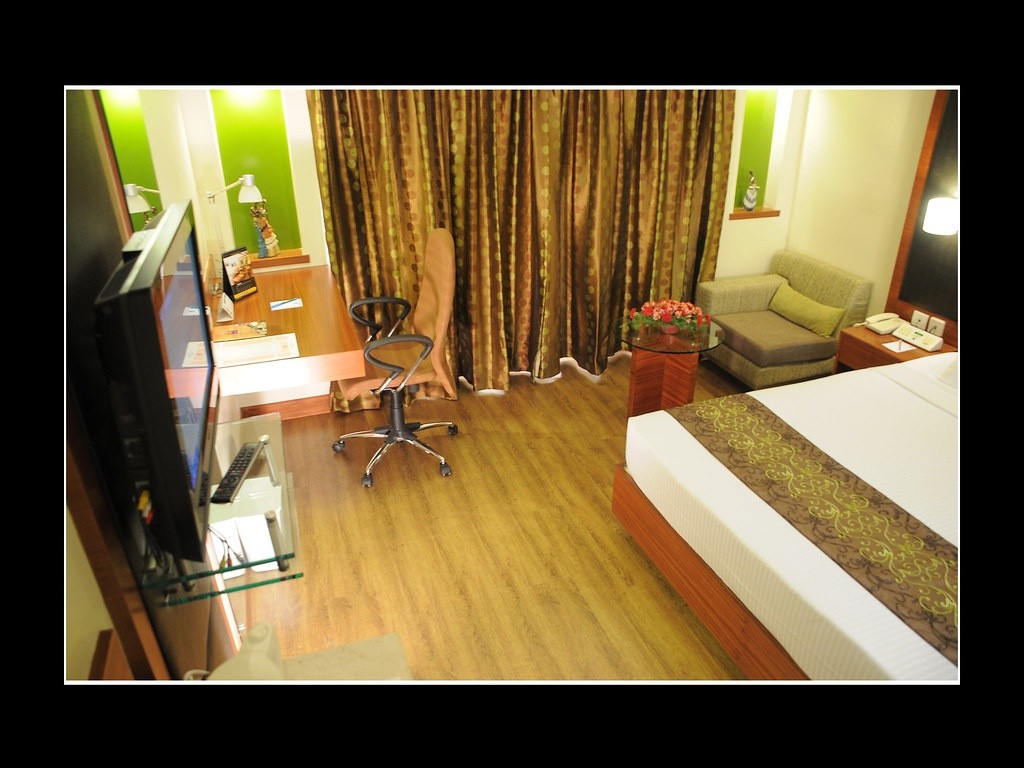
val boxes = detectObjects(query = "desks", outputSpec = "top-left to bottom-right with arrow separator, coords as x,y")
210,264 -> 368,396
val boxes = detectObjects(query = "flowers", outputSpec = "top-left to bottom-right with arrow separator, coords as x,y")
625,301 -> 712,339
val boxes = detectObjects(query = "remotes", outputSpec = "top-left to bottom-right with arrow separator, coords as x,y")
210,442 -> 263,503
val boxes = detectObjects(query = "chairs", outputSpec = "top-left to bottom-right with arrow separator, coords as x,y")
330,227 -> 460,488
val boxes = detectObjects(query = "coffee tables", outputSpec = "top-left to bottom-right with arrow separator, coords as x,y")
612,315 -> 726,420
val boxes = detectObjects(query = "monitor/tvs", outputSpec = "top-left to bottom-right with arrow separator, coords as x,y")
95,195 -> 220,564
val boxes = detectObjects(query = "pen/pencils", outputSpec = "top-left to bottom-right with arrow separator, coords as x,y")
269,297 -> 299,310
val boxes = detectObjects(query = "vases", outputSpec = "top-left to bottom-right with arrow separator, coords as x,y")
658,321 -> 683,335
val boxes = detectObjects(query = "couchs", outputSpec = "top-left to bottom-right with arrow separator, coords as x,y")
695,250 -> 876,393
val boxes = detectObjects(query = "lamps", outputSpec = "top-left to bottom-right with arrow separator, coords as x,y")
209,174 -> 266,290
920,197 -> 960,236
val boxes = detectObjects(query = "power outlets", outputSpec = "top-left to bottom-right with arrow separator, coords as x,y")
927,317 -> 946,337
911,310 -> 930,331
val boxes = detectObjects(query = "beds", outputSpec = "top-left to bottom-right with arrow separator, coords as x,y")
609,350 -> 960,681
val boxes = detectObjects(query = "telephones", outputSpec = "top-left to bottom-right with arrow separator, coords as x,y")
863,312 -> 909,336
